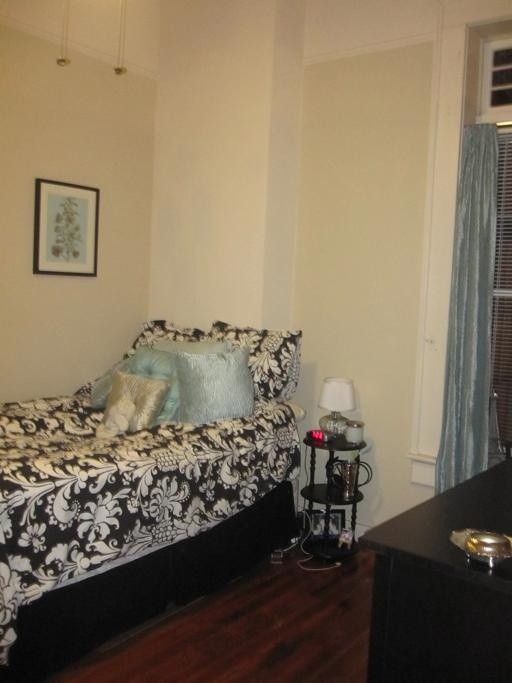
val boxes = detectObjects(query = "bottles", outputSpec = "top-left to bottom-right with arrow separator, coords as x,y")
345,421 -> 365,443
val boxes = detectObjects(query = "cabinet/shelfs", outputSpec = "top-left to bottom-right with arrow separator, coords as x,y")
300,433 -> 367,561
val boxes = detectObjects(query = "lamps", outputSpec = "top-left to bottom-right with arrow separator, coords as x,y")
317,377 -> 356,435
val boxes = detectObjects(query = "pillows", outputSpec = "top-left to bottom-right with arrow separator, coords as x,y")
75,319 -> 301,430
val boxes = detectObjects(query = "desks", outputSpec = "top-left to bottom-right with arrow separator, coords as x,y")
358,456 -> 512,682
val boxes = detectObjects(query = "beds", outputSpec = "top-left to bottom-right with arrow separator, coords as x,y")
0,395 -> 299,683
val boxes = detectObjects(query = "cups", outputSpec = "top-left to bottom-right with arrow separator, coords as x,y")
341,462 -> 359,501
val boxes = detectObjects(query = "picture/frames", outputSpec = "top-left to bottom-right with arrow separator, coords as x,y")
306,509 -> 345,542
33,178 -> 100,277
